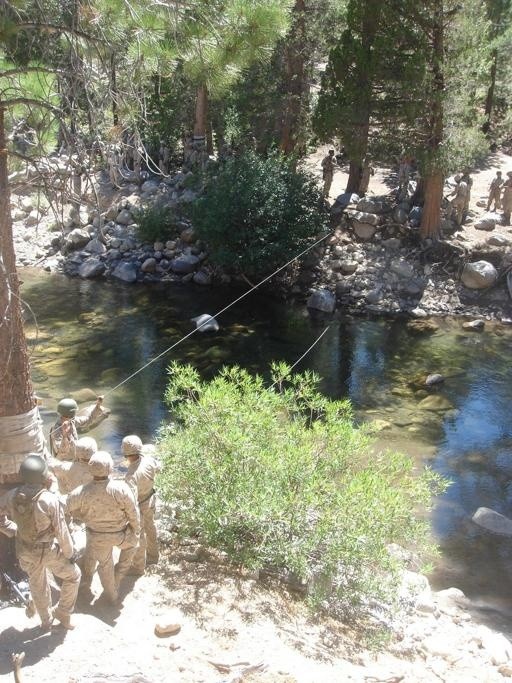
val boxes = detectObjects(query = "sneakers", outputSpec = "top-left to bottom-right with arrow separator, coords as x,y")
42,612 -> 54,634
53,610 -> 75,630
78,557 -> 160,606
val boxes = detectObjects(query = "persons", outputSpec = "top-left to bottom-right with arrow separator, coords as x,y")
322,150 -> 337,193
91,131 -> 258,188
448,167 -> 512,226
358,153 -> 374,196
398,149 -> 416,189
3,394 -> 159,631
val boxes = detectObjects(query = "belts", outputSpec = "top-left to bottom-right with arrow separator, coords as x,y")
33,541 -> 51,549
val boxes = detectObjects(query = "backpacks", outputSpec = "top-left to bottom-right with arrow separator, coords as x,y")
12,486 -> 54,545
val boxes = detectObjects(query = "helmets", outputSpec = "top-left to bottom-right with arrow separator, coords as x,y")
19,455 -> 48,484
89,451 -> 114,477
453,170 -> 512,184
74,437 -> 98,460
121,435 -> 144,456
57,398 -> 78,417
